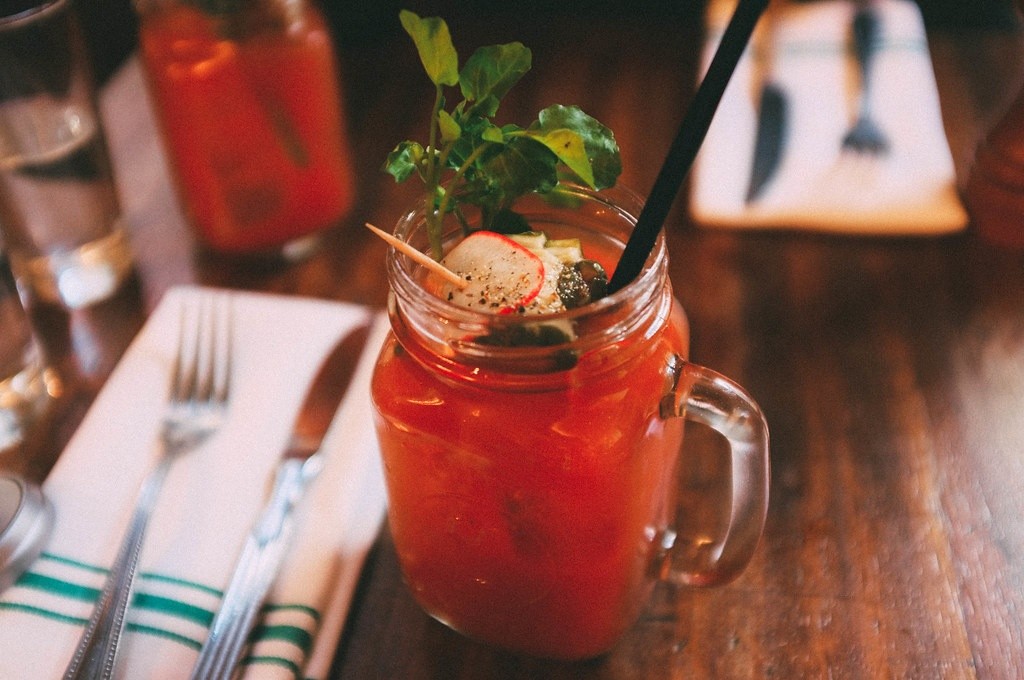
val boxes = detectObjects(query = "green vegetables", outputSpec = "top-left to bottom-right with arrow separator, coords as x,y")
379,7 -> 624,261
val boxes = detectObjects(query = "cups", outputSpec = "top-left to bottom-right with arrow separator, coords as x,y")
371,168 -> 772,657
1,1 -> 136,326
137,1 -> 360,267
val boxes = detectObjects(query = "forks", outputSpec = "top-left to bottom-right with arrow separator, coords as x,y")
59,307 -> 236,680
836,9 -> 893,177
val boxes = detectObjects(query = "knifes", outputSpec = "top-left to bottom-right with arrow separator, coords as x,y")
744,14 -> 800,207
187,325 -> 375,680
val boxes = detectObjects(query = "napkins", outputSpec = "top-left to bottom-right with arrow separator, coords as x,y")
686,0 -> 970,236
0,283 -> 386,680
